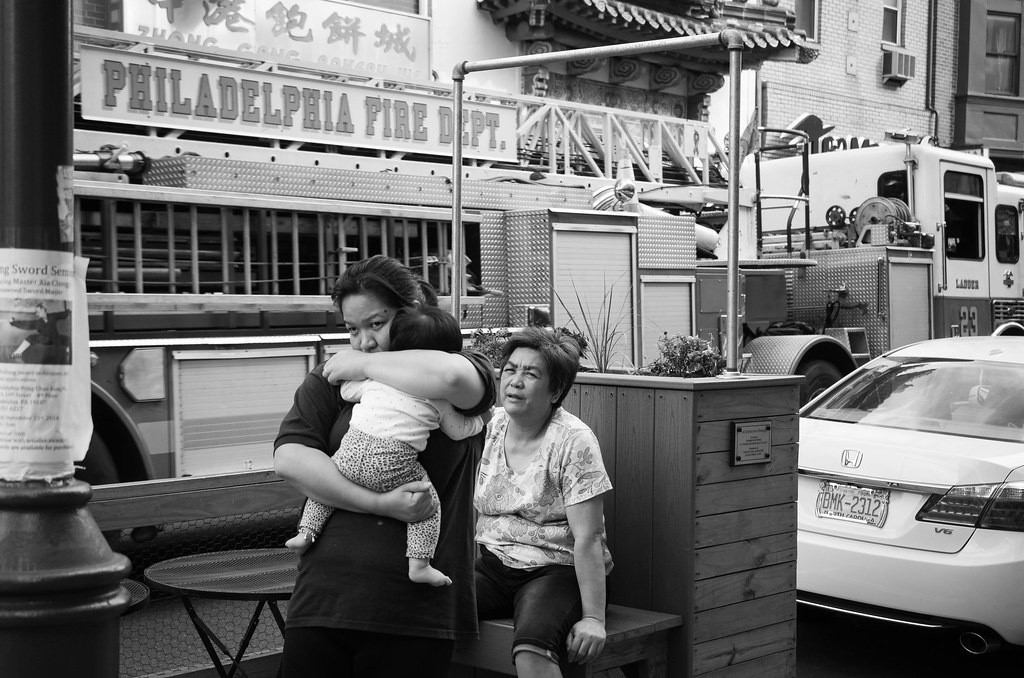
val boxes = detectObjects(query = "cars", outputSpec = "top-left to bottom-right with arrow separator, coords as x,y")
793,333 -> 1024,657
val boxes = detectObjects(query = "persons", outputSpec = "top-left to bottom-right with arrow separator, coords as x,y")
286,305 -> 495,585
475,328 -> 613,678
7,302 -> 72,359
272,254 -> 496,678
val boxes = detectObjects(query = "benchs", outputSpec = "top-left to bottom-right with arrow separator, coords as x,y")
453,601 -> 683,678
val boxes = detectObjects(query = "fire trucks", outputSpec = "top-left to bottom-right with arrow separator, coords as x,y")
73,22 -> 1021,538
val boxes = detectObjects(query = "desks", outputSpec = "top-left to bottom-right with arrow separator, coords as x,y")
145,548 -> 300,678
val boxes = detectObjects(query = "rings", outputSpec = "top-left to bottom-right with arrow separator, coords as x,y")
323,368 -> 329,374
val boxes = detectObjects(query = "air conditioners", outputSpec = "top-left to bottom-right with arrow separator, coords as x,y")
883,52 -> 916,80
